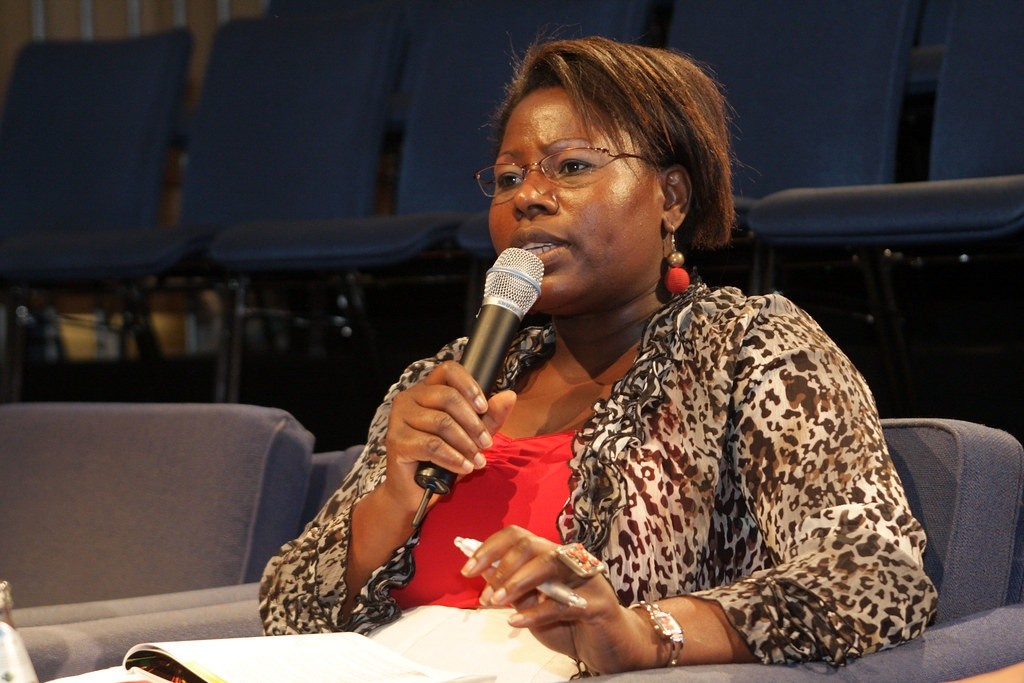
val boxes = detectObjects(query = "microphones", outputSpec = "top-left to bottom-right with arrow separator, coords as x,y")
414,247 -> 546,495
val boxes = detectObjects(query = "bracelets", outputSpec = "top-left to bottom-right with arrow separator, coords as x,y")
628,600 -> 685,668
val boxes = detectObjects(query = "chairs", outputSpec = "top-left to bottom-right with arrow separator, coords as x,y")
0,396 -> 1024,683
0,0 -> 1024,405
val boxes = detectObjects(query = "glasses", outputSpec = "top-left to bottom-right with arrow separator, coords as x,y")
475,147 -> 671,198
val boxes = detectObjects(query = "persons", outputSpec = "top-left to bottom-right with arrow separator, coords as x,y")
258,36 -> 941,673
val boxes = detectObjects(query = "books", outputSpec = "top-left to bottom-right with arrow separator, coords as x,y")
43,631 -> 448,683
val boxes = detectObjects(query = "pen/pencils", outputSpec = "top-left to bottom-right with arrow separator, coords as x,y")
454,536 -> 588,611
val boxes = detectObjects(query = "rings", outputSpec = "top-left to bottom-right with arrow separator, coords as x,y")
555,543 -> 605,581
